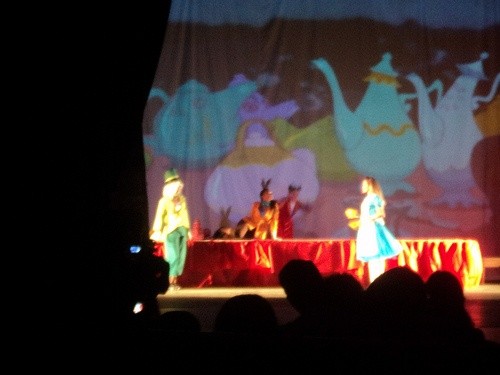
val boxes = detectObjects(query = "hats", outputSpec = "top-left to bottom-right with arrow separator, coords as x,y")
163,168 -> 179,184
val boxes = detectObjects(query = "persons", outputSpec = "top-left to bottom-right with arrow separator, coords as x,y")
235,188 -> 284,240
278,183 -> 324,240
155,261 -> 500,375
150,170 -> 193,291
356,176 -> 403,283
